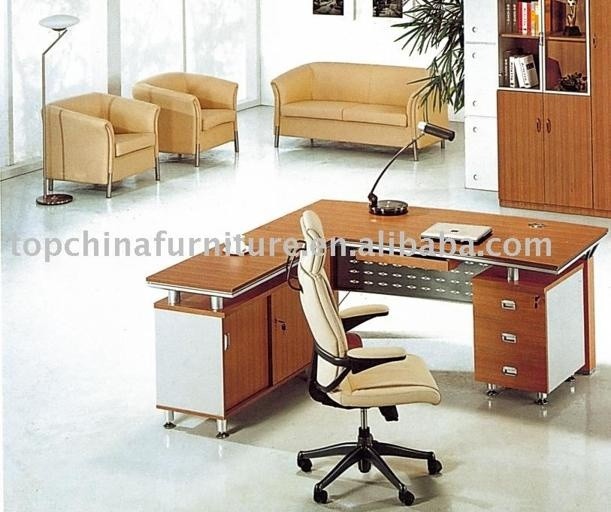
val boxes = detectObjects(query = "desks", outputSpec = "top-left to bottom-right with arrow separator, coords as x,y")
146,198 -> 608,439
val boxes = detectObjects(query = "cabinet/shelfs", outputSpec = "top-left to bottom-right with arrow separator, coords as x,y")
499,0 -> 591,96
589,0 -> 611,211
497,90 -> 594,209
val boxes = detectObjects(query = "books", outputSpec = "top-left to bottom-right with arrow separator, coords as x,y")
503,0 -> 566,90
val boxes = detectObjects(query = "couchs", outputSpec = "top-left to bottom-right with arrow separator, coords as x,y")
271,62 -> 449,161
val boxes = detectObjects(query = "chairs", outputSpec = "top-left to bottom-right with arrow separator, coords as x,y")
132,72 -> 239,166
40,92 -> 160,197
285,209 -> 442,506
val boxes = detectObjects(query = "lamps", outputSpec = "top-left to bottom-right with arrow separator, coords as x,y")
36,15 -> 79,205
368,121 -> 455,217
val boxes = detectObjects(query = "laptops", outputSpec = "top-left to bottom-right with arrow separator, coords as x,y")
420,222 -> 493,245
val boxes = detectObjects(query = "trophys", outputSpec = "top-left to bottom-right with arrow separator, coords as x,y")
563,0 -> 582,36
